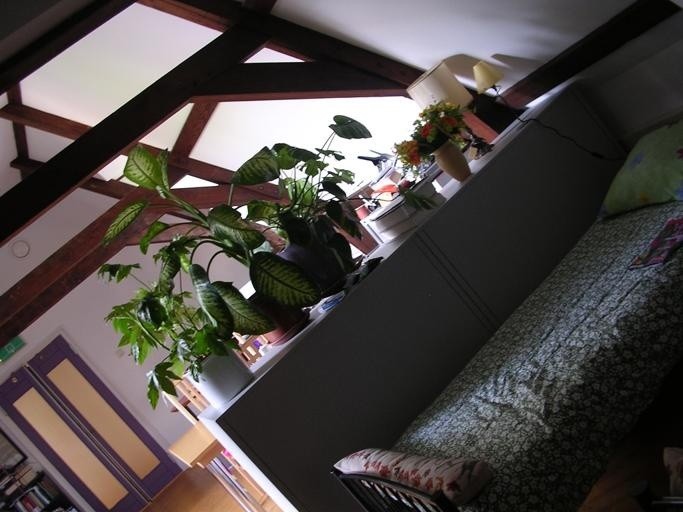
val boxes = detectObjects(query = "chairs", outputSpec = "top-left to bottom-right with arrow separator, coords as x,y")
228,332 -> 269,369
158,375 -> 210,425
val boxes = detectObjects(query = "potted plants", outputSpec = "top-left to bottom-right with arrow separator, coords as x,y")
103,115 -> 436,335
96,261 -> 255,409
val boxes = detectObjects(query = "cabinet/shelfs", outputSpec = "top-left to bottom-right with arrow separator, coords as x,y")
0,470 -> 80,512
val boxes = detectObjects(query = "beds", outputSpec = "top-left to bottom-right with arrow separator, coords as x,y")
330,116 -> 683,507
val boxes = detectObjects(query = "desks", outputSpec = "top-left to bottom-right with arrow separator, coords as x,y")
164,390 -> 205,422
167,419 -> 263,512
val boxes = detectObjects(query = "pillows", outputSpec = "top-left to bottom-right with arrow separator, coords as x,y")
593,119 -> 683,223
330,446 -> 493,506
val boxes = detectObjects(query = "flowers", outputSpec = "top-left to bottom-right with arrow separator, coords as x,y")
393,99 -> 465,177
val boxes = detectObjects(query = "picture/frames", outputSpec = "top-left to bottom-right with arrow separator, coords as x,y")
0,426 -> 28,473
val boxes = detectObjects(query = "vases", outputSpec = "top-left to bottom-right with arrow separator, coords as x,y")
429,139 -> 471,182
354,203 -> 370,220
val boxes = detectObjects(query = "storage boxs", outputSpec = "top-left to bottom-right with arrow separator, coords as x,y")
367,167 -> 400,193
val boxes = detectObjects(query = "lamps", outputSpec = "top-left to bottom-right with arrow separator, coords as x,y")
472,61 -> 519,120
406,61 -> 499,144
